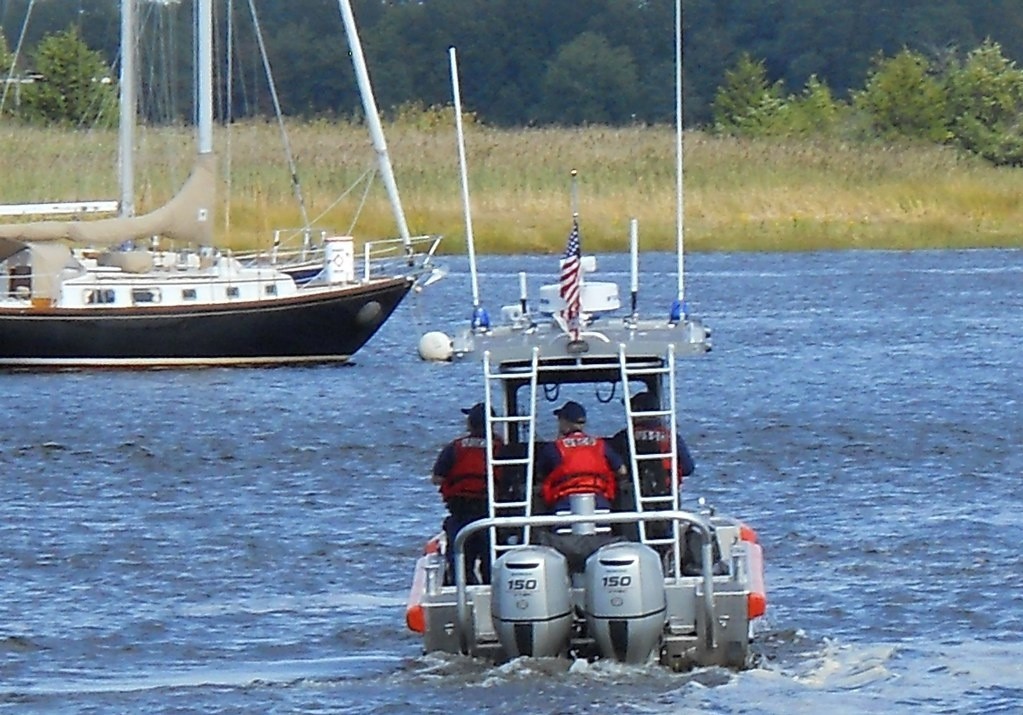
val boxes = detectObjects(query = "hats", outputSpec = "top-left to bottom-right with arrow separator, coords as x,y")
553,401 -> 586,423
461,402 -> 496,427
621,392 -> 660,411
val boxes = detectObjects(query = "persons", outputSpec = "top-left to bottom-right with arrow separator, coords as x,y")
615,392 -> 694,531
538,401 -> 626,535
432,402 -> 511,585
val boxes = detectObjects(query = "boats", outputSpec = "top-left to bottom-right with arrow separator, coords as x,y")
418,1 -> 711,361
405,167 -> 766,674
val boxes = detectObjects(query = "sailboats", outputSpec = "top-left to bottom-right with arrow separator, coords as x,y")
1,0 -> 447,367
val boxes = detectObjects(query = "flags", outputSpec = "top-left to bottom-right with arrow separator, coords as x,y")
560,223 -> 582,319
